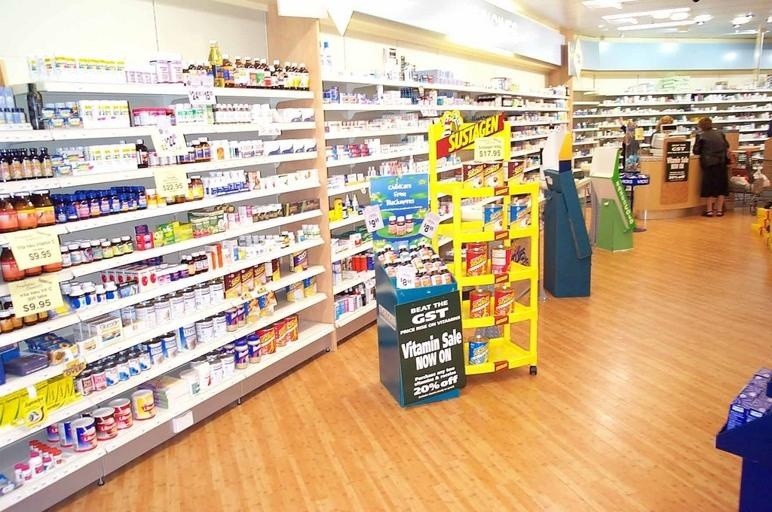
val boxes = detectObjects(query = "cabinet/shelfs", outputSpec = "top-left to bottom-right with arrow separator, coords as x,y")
323,84 -> 570,345
424,109 -> 544,380
573,68 -> 772,220
2,58 -> 335,512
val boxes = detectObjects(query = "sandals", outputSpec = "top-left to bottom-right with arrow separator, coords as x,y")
716,210 -> 724,217
701,210 -> 714,217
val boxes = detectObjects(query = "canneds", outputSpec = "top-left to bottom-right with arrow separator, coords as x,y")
256,313 -> 299,355
461,159 -> 529,365
47,389 -> 155,452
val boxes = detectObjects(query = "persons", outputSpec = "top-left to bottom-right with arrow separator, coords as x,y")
648,115 -> 673,147
750,120 -> 771,217
691,117 -> 730,218
618,117 -> 639,169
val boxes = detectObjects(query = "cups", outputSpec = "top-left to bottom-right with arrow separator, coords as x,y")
377,86 -> 432,177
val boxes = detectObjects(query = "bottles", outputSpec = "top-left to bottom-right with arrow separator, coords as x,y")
325,82 -> 376,318
388,213 -> 415,236
376,241 -> 454,291
476,95 -> 515,108
0,41 -> 326,497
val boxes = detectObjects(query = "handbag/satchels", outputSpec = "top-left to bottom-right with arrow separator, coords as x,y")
720,134 -> 737,165
